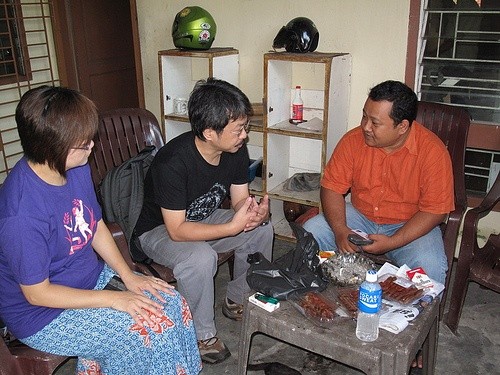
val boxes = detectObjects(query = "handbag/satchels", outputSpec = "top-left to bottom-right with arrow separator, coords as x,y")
245,218 -> 329,300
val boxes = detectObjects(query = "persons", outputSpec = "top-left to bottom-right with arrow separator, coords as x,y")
0,85 -> 203,375
302,80 -> 456,303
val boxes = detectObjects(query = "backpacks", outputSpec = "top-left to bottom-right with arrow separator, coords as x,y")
101,144 -> 164,261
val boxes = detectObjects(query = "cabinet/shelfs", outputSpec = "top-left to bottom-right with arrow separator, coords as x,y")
156,46 -> 354,243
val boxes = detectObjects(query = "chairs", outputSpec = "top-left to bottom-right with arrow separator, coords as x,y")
352,100 -> 500,335
0,108 -> 235,375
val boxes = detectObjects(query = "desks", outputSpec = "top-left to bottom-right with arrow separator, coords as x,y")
236,272 -> 440,375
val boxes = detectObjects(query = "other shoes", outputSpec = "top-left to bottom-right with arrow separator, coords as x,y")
198,336 -> 231,363
222,297 -> 245,320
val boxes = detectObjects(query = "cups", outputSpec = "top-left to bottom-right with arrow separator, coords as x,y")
173,97 -> 186,115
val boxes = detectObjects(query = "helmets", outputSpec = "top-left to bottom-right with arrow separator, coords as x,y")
272,17 -> 320,53
172,6 -> 217,50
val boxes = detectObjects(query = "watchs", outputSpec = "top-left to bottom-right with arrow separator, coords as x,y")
260,217 -> 269,226
132,77 -> 274,365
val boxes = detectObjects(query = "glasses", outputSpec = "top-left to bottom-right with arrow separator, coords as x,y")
71,140 -> 92,150
223,119 -> 252,136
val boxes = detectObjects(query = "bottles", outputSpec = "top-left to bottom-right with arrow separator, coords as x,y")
292,85 -> 303,123
355,270 -> 381,342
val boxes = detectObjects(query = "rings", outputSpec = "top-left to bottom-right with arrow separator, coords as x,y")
156,280 -> 158,284
